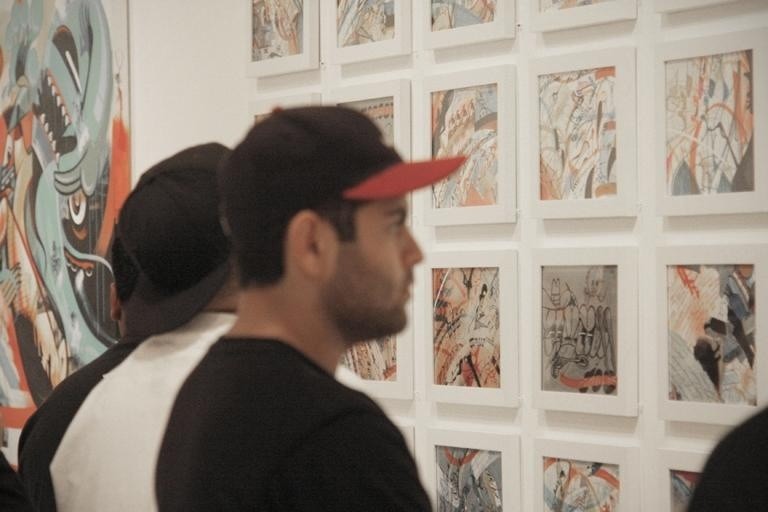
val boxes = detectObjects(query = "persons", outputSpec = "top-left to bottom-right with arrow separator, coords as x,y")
45,139 -> 237,512
17,235 -> 138,511
155,102 -> 470,511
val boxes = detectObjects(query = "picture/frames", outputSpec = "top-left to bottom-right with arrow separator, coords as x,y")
412,63 -> 517,226
413,425 -> 523,512
318,2 -> 412,65
654,25 -> 767,219
523,437 -> 636,511
641,445 -> 710,511
244,1 -> 320,77
244,90 -> 320,134
528,1 -> 639,33
410,1 -> 516,50
653,2 -> 734,19
525,46 -> 638,220
323,77 -> 411,211
333,277 -> 415,401
652,244 -> 767,426
527,245 -> 639,419
412,249 -> 521,411
390,423 -> 415,457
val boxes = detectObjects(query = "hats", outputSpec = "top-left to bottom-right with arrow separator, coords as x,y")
118,143 -> 232,337
216,106 -> 467,236
